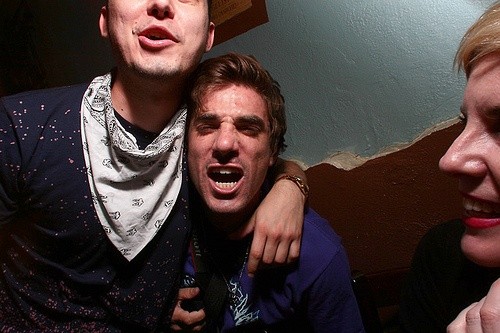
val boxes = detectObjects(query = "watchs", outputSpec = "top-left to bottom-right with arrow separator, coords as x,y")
275,174 -> 310,202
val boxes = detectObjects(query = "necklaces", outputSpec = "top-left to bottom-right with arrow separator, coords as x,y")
202,230 -> 255,311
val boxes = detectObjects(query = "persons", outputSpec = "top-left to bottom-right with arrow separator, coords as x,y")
169,52 -> 365,333
384,0 -> 500,333
0,0 -> 311,333
